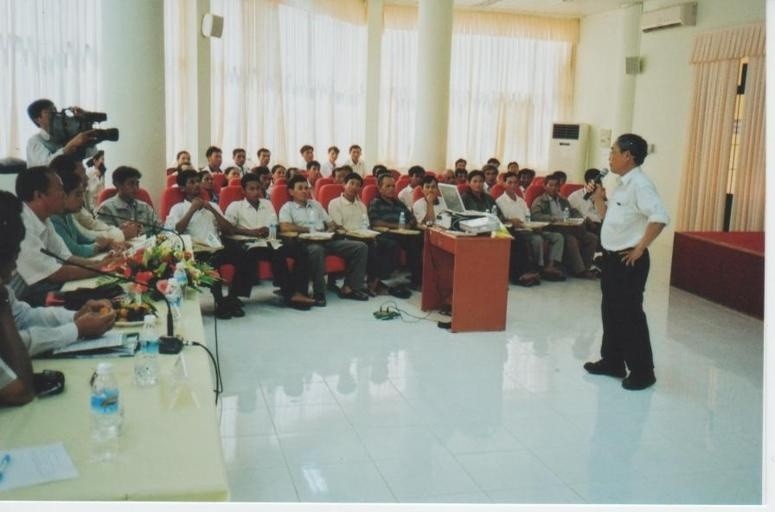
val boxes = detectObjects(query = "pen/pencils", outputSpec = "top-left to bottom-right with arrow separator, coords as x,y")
0,454 -> 11,481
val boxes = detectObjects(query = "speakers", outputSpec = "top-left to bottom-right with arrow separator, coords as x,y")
202,13 -> 224,39
626,56 -> 640,74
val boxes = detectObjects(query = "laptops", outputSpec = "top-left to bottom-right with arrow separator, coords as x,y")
438,183 -> 491,216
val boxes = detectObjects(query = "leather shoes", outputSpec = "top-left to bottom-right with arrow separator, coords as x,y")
584,358 -> 626,378
622,367 -> 655,390
285,288 -> 325,307
517,268 -> 598,285
216,296 -> 244,318
339,284 -> 376,300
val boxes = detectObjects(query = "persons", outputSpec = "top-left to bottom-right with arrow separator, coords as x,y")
583,133 -> 668,392
164,144 -> 607,319
1,98 -> 158,410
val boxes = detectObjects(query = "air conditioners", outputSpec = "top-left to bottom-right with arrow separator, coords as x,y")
547,123 -> 590,182
642,4 -> 697,33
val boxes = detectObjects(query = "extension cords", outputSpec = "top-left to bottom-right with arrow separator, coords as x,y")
374,310 -> 400,320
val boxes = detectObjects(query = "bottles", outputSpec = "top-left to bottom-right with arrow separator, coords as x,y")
360,213 -> 368,235
163,215 -> 175,237
399,211 -> 406,233
486,204 -> 498,217
135,263 -> 189,387
563,207 -> 570,224
524,207 -> 530,224
269,221 -> 277,241
89,362 -> 123,440
309,210 -> 317,236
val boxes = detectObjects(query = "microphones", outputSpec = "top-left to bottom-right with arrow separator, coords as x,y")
97,212 -> 185,251
40,248 -> 182,353
583,167 -> 608,200
